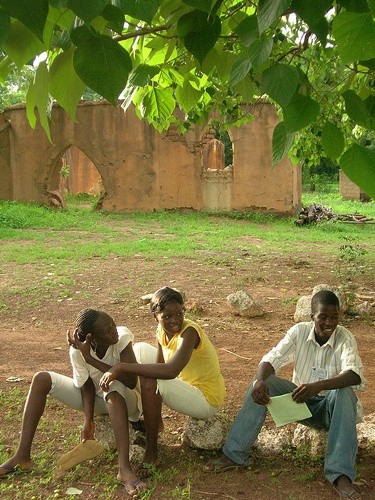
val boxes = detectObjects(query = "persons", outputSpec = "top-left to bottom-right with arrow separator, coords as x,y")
203,290 -> 368,500
0,309 -> 147,498
98,287 -> 226,479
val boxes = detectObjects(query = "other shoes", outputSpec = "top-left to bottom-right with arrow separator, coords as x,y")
131,454 -> 160,478
58,440 -> 104,471
129,418 -> 145,432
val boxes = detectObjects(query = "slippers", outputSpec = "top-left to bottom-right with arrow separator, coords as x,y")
0,464 -> 31,478
332,483 -> 362,500
117,473 -> 145,497
202,458 -> 246,473
